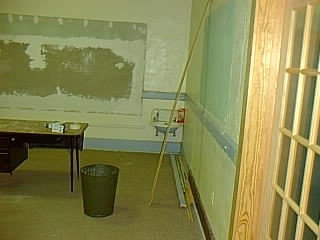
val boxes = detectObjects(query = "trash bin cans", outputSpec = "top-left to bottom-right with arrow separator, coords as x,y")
79,163 -> 122,218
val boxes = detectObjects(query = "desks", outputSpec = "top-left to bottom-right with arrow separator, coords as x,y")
0,119 -> 88,192
